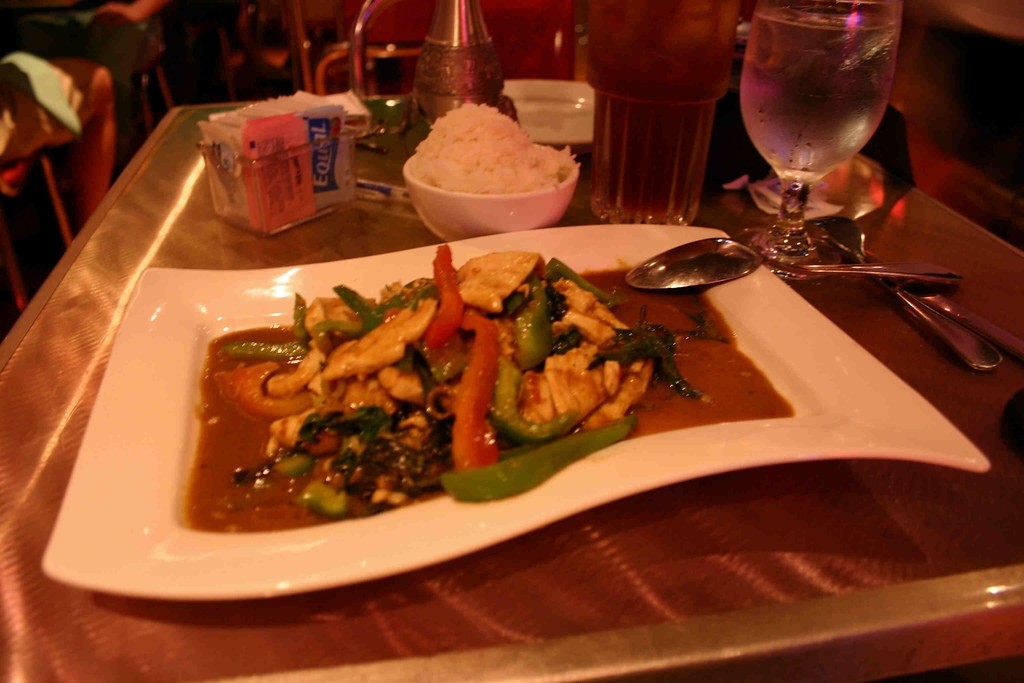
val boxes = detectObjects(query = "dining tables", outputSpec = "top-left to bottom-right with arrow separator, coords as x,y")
0,102 -> 1024,683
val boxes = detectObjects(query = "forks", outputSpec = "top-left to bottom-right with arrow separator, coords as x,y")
805,213 -> 1003,372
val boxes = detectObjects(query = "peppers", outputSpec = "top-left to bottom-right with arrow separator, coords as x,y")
221,244 -> 637,521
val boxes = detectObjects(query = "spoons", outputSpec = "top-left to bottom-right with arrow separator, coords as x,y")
625,237 -> 965,292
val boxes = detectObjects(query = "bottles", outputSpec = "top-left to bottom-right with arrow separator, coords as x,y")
407,0 -> 504,141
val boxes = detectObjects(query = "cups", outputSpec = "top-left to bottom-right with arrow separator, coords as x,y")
584,0 -> 742,223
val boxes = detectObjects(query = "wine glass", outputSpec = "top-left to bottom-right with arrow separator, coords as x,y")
730,1 -> 904,280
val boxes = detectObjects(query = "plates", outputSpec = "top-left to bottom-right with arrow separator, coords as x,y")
498,78 -> 595,156
41,222 -> 991,603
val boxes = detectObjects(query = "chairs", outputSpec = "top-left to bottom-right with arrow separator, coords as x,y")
293,0 -> 422,96
349,0 -> 576,98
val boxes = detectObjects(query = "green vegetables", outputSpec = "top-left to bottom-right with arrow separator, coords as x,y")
298,283 -> 730,512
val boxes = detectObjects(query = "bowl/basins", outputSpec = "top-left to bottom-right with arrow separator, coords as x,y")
402,144 -> 580,242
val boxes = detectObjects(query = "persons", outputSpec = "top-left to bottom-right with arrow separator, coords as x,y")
0,0 -> 238,233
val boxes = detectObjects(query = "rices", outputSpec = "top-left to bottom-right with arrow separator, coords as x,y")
410,102 -> 582,196
